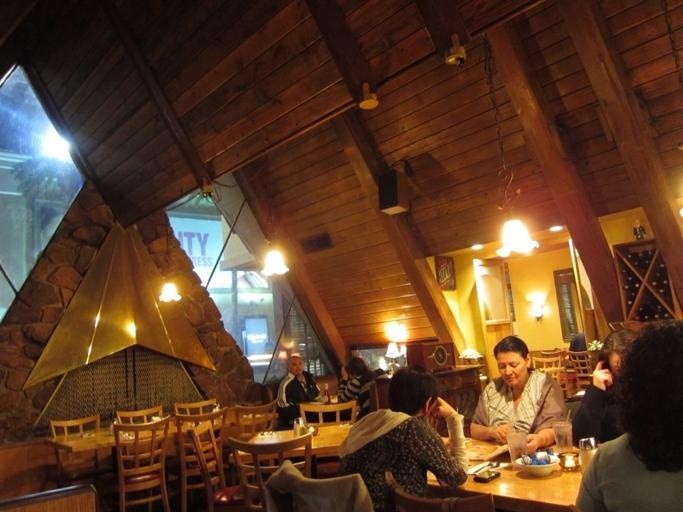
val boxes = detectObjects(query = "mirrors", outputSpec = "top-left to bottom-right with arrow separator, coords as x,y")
475,261 -> 518,328
552,267 -> 582,342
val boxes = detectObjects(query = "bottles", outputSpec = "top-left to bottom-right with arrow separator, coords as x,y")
110,419 -> 120,437
580,436 -> 594,474
320,383 -> 331,404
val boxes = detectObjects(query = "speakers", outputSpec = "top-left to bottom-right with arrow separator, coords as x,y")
405,342 -> 456,373
379,170 -> 410,215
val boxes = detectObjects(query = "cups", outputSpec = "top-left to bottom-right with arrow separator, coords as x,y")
558,450 -> 580,473
330,395 -> 337,404
550,420 -> 573,460
506,429 -> 528,473
293,417 -> 318,437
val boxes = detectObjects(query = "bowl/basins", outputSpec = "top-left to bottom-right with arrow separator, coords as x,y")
514,454 -> 561,477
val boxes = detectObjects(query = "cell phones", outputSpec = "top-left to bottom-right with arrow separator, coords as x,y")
473,471 -> 500,483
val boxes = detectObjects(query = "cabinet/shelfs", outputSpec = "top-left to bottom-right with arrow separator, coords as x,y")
611,238 -> 681,323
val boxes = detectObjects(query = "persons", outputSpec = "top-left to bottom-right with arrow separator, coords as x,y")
469,334 -> 568,454
275,355 -> 322,432
632,219 -> 646,240
574,318 -> 682,511
337,364 -> 470,512
336,356 -> 378,421
571,328 -> 639,450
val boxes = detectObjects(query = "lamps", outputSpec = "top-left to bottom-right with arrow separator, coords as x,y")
144,210 -> 185,303
248,164 -> 289,278
473,33 -> 542,260
